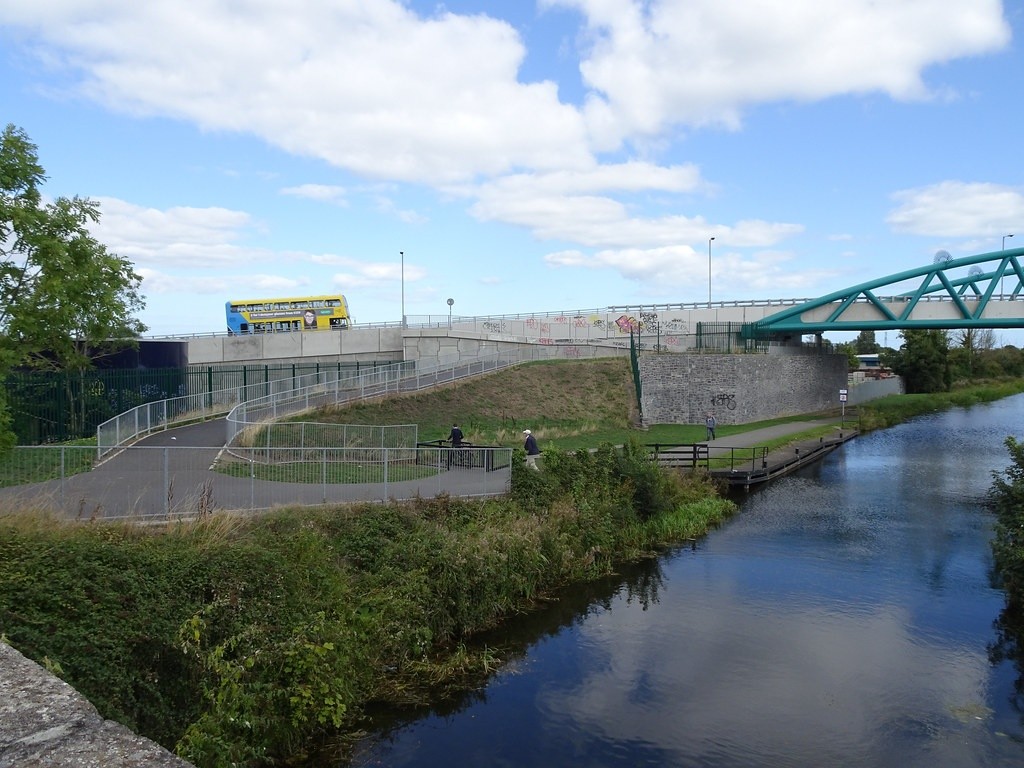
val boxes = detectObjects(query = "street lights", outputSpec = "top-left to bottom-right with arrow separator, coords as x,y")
400,252 -> 403,321
1001,235 -> 1014,300
709,237 -> 715,308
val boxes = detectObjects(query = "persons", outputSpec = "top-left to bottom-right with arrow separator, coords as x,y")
304,309 -> 317,329
523,430 -> 541,472
447,424 -> 464,466
705,413 -> 716,441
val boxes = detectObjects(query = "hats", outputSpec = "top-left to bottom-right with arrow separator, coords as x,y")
453,424 -> 457,427
523,429 -> 531,435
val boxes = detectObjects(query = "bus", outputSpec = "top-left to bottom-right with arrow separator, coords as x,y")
225,295 -> 352,337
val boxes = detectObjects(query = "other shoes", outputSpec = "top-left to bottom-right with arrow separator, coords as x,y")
706,439 -> 709,441
713,438 -> 715,440
